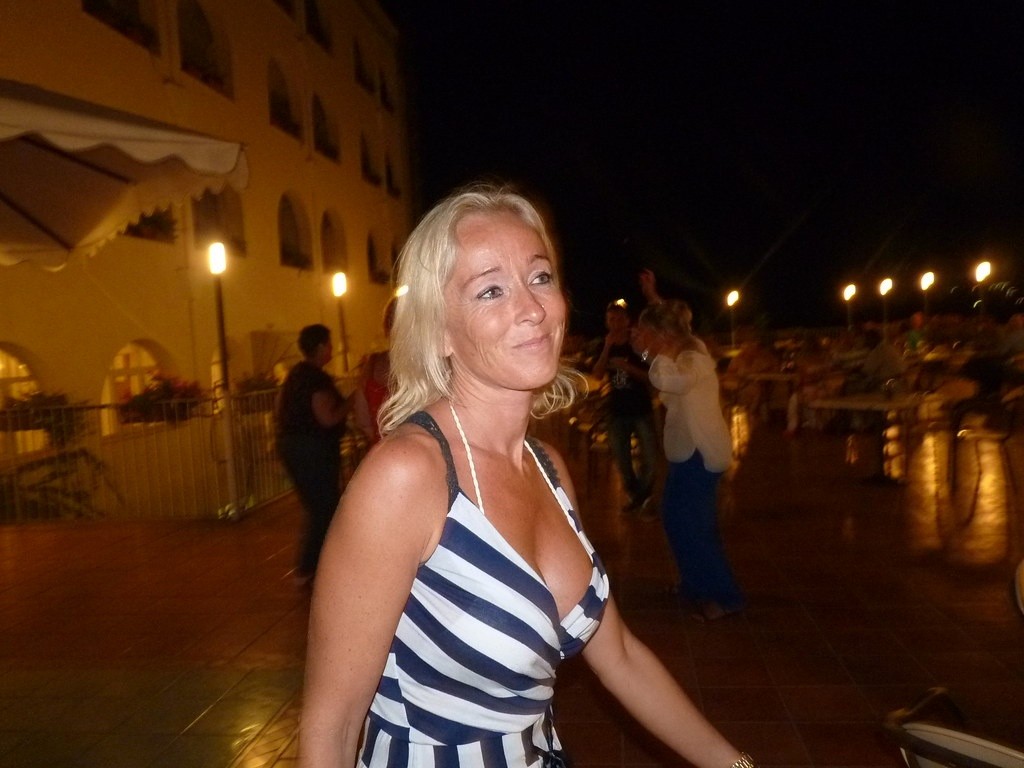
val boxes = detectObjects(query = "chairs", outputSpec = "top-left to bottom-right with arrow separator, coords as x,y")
881,683 -> 1024,768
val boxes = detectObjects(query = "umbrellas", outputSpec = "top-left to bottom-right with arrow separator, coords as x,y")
0,74 -> 253,270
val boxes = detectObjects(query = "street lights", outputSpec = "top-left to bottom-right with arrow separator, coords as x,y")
843,284 -> 856,335
878,278 -> 892,342
207,239 -> 242,525
331,271 -> 348,377
920,271 -> 934,334
726,289 -> 741,353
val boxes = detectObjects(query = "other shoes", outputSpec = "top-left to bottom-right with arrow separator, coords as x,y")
662,581 -> 690,603
692,599 -> 734,623
621,499 -> 641,515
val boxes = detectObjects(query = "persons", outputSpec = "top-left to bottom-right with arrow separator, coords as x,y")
727,315 -> 1024,487
589,268 -> 744,620
277,325 -> 358,583
298,187 -> 758,768
355,298 -> 397,449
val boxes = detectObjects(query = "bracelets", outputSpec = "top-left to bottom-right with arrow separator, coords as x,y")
730,753 -> 758,768
